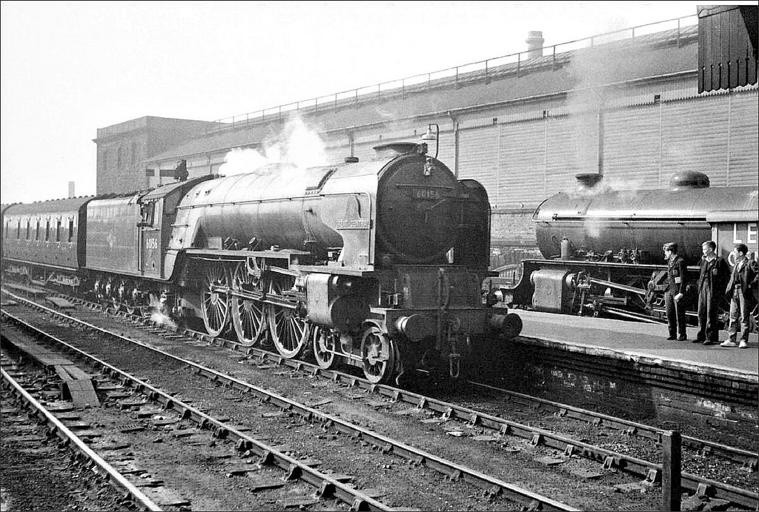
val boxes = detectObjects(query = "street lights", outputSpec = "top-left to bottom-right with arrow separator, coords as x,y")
422,122 -> 440,160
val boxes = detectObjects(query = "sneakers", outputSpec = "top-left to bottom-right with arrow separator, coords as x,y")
667,335 -> 748,348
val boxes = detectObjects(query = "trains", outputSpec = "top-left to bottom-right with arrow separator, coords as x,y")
492,171 -> 758,334
0,141 -> 521,388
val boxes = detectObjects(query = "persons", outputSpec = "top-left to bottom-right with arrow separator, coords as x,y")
663,241 -> 690,341
691,240 -> 731,345
719,244 -> 758,348
